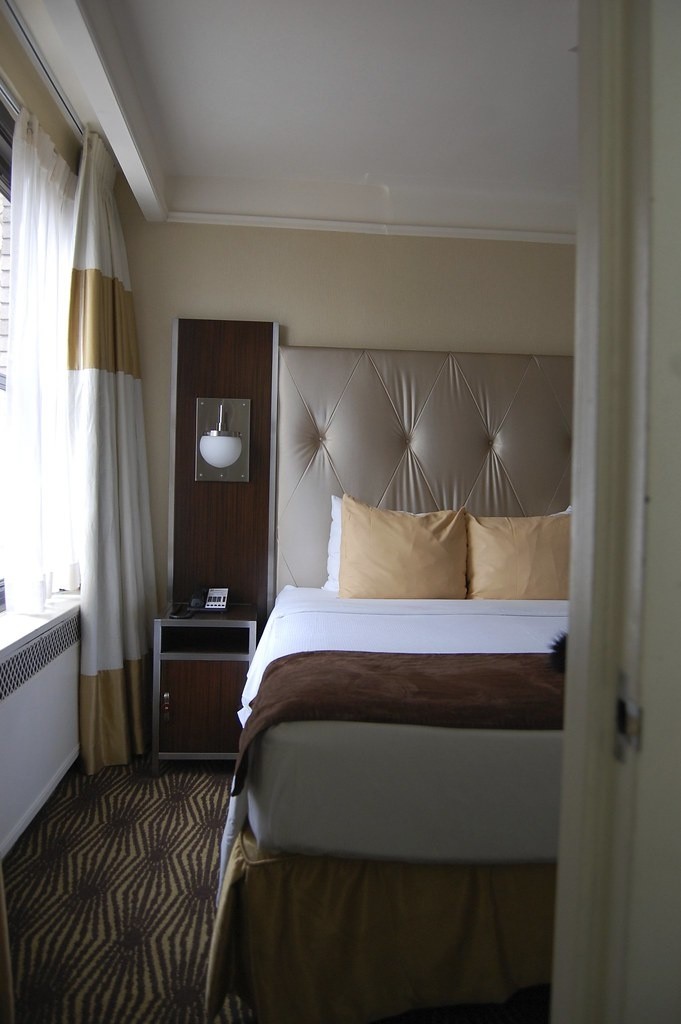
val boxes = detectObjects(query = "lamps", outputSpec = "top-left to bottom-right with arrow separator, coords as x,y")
199,403 -> 243,468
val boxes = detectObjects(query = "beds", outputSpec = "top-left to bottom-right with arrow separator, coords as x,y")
200,347 -> 579,1024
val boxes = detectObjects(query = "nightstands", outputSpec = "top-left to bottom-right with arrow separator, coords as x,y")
152,607 -> 258,771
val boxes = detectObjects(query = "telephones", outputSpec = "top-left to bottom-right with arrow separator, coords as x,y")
187,585 -> 231,612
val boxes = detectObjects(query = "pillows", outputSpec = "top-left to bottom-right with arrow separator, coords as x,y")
320,493 -> 570,599
